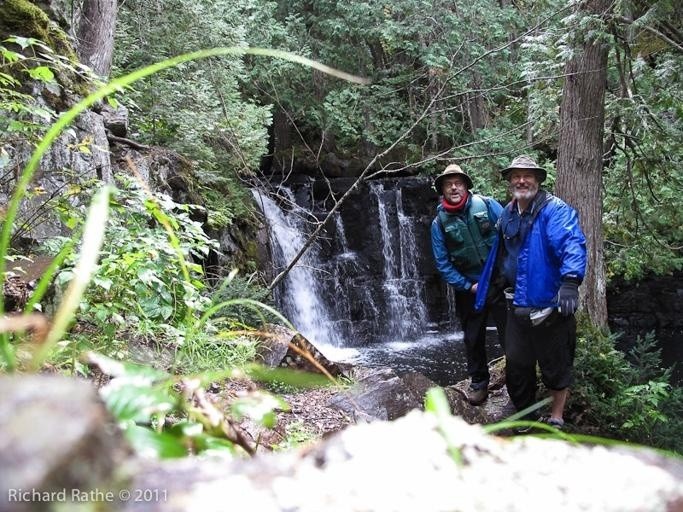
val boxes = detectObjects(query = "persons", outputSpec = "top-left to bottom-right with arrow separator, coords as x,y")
432,156 -> 588,436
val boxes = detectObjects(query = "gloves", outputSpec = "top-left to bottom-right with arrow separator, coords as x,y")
551,282 -> 579,317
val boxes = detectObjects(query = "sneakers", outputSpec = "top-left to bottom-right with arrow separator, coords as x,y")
469,375 -> 489,404
512,408 -> 543,435
547,417 -> 565,433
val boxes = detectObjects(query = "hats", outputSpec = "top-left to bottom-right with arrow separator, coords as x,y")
501,154 -> 547,185
435,163 -> 473,193
